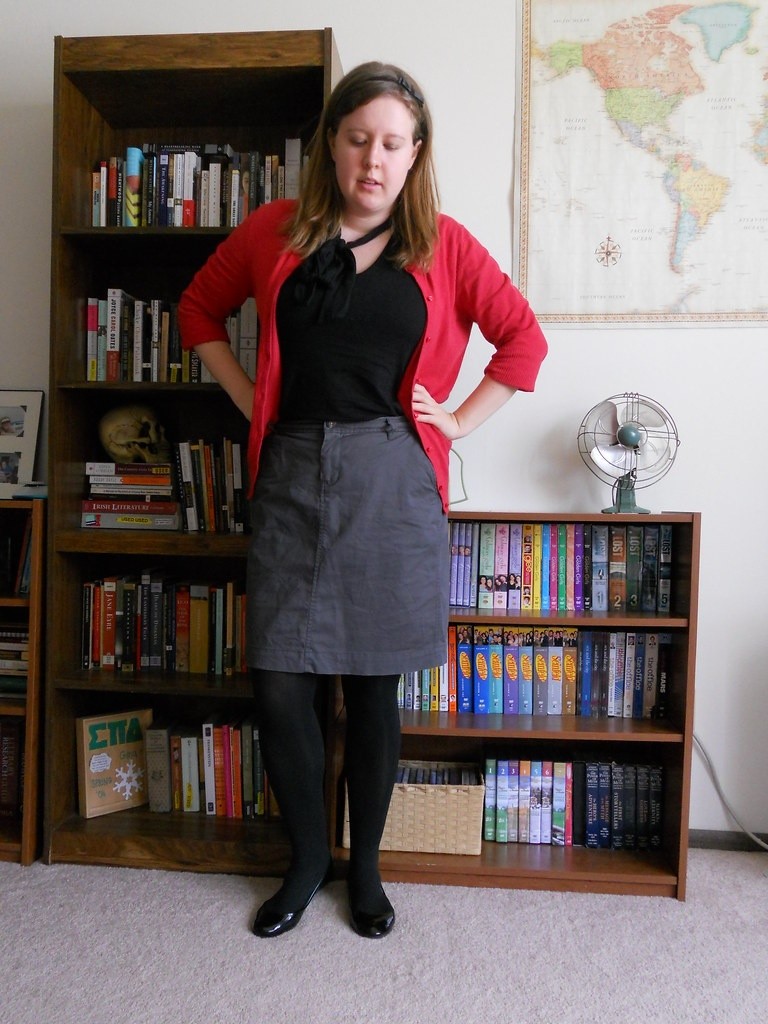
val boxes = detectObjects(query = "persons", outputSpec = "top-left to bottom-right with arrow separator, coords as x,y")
0,416 -> 16,435
1,460 -> 12,478
172,61 -> 549,938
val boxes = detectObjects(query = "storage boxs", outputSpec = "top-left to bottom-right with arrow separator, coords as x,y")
342,760 -> 485,856
76,707 -> 153,818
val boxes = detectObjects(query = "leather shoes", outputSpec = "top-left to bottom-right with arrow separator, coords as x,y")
253,856 -> 334,937
345,862 -> 394,939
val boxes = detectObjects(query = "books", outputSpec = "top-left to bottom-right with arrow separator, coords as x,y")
86,133 -> 313,387
72,437 -> 278,820
0,509 -> 34,846
347,518 -> 684,852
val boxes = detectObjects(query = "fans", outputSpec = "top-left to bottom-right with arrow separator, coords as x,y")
577,392 -> 681,514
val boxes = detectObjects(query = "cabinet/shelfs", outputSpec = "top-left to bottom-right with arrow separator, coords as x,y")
0,499 -> 46,865
44,29 -> 701,902
